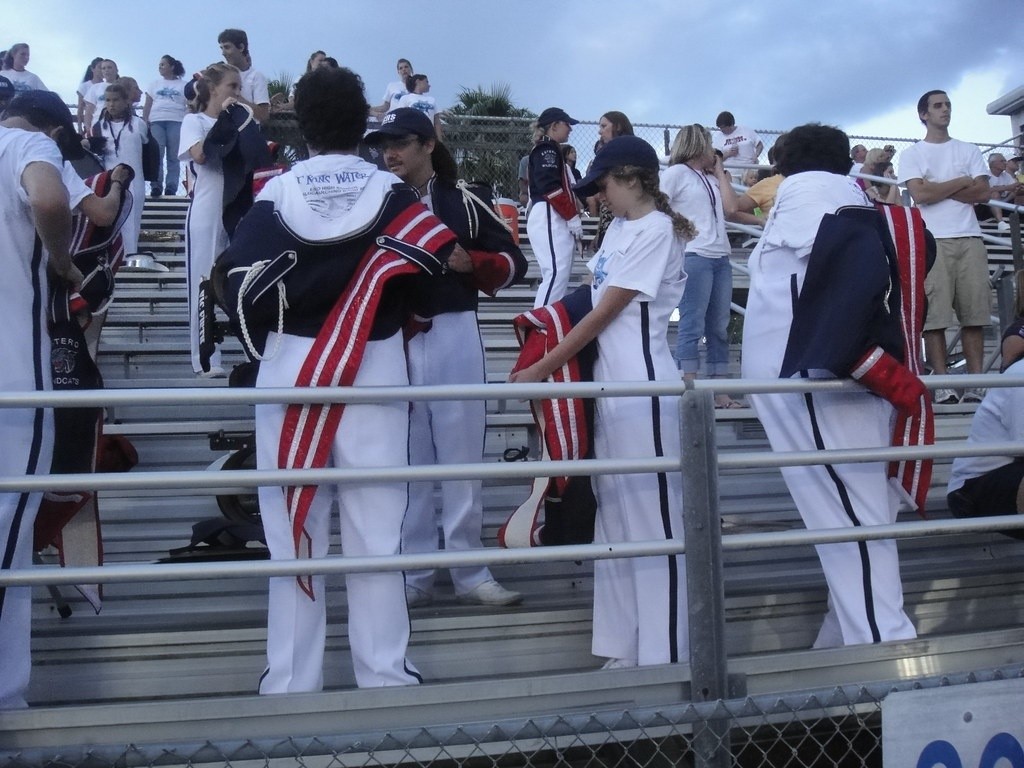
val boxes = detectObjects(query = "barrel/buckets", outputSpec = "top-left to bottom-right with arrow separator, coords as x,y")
491,198 -> 520,247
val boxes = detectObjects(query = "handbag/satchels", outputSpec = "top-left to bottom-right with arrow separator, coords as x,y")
142,122 -> 160,182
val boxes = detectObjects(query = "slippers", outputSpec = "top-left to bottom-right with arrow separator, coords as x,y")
713,398 -> 743,410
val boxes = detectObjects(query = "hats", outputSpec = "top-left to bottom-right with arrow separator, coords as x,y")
865,147 -> 893,168
363,108 -> 437,147
0,75 -> 15,96
1004,154 -> 1024,163
883,145 -> 895,155
536,107 -> 580,125
572,134 -> 659,197
202,111 -> 238,157
12,90 -> 82,144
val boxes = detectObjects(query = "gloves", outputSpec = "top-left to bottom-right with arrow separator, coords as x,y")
567,213 -> 583,241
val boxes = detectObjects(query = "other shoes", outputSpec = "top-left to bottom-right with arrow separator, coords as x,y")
402,583 -> 434,609
455,581 -> 523,606
165,190 -> 175,195
151,187 -> 162,196
196,365 -> 229,379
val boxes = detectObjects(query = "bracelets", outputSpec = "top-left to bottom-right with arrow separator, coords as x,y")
111,179 -> 124,187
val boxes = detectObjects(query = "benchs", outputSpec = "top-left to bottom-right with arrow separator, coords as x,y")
0,194 -> 1024,768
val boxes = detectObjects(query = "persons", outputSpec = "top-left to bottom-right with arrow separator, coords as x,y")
0,28 -> 1024,709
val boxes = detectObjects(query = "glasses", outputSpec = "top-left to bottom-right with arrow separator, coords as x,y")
926,101 -> 951,109
372,136 -> 421,154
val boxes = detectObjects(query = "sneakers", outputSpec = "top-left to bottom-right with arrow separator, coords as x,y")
934,386 -> 959,404
998,221 -> 1010,230
958,390 -> 984,403
